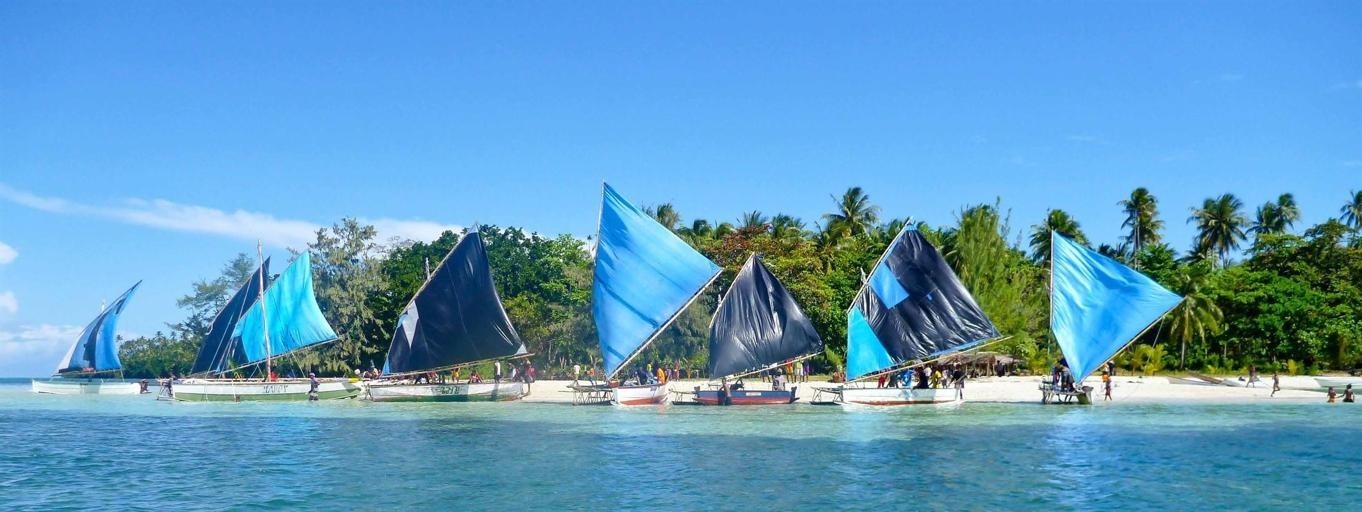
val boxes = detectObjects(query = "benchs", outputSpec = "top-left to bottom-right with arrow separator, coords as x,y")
1040,380 -> 1091,404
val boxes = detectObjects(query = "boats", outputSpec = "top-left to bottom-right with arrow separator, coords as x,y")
1185,367 -> 1225,384
1163,372 -> 1210,385
1223,378 -> 1269,388
1314,375 -> 1362,392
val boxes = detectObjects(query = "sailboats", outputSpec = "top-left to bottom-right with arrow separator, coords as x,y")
815,216 -> 1016,405
155,240 -> 358,401
359,222 -> 538,406
562,178 -> 725,406
673,248 -> 824,410
1037,226 -> 1190,407
31,276 -> 147,395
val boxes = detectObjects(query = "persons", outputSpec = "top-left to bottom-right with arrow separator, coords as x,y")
996,360 -> 1003,376
1270,370 -> 1280,397
1338,384 -> 1354,402
832,368 -> 846,383
1101,359 -> 1115,401
1245,364 -> 1255,387
588,364 -> 594,386
762,357 -> 810,390
620,355 -> 680,386
364,360 -> 540,395
308,372 -> 320,399
594,363 -> 600,377
877,357 -> 964,399
1051,353 -> 1076,402
1326,387 -> 1336,403
271,368 -> 278,382
571,360 -> 581,385
720,378 -> 731,406
